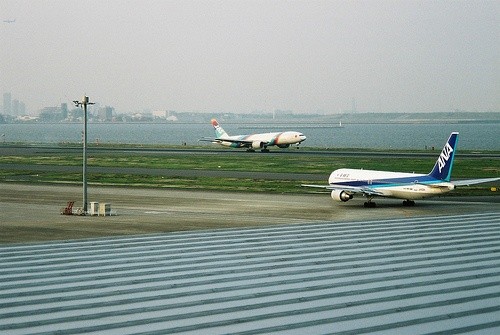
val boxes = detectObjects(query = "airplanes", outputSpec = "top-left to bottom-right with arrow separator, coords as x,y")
300,132 -> 500,208
199,118 -> 308,152
